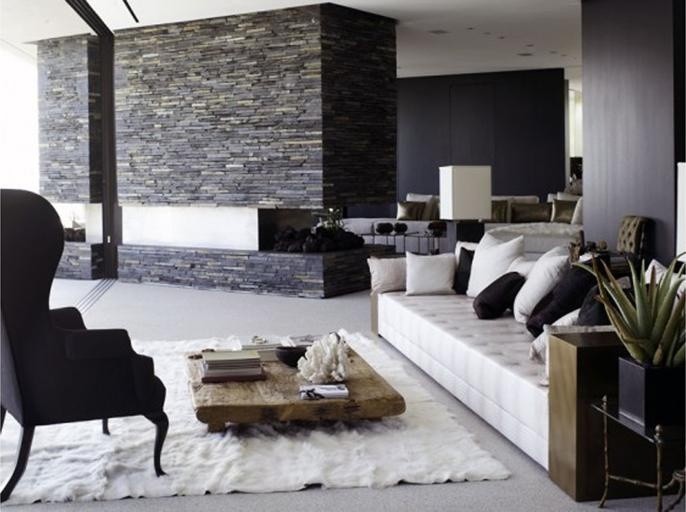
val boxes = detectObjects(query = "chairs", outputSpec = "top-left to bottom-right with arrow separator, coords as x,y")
585,210 -> 661,280
0,184 -> 171,504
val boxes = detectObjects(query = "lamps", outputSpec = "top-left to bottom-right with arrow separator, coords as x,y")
438,164 -> 495,257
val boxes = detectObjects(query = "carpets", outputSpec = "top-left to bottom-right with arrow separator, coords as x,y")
0,330 -> 520,508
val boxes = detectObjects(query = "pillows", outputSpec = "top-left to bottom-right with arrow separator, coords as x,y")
392,191 -> 583,229
453,233 -> 630,387
405,251 -> 457,296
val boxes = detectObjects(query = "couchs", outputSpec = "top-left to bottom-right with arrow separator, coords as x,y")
334,215 -> 584,241
364,254 -> 686,502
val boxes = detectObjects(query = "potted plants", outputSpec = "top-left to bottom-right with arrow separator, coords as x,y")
572,247 -> 686,444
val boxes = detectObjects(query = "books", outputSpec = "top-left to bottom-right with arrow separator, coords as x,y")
280,333 -> 325,349
199,349 -> 265,384
298,383 -> 349,401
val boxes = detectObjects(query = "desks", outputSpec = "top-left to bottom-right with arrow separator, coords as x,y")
588,391 -> 686,511
